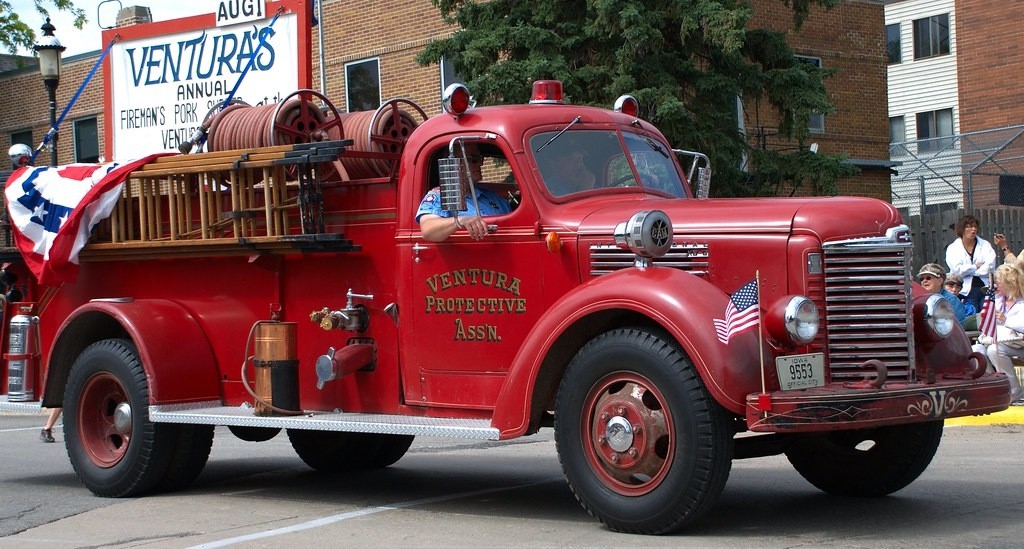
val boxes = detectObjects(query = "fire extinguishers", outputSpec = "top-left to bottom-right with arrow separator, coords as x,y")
252,301 -> 303,416
6,305 -> 40,402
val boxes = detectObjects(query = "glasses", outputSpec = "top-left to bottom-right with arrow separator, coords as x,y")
460,154 -> 484,165
946,280 -> 963,287
920,276 -> 936,281
966,225 -> 978,229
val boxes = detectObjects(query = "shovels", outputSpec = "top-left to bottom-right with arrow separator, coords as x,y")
81,138 -> 364,264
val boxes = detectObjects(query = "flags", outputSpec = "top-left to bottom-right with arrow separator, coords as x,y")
713,280 -> 759,345
977,284 -> 996,340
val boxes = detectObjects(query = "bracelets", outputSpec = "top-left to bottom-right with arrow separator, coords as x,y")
1002,247 -> 1009,251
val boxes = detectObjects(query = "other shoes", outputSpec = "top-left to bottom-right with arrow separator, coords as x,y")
1011,387 -> 1024,402
39,428 -> 55,443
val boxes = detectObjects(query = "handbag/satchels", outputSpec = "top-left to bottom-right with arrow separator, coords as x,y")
978,324 -> 1024,349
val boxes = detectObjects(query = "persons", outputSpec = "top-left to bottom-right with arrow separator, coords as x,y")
544,138 -> 596,199
916,215 -> 1024,405
414,142 -> 511,242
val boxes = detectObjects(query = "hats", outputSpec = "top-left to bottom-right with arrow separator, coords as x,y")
945,273 -> 964,287
916,263 -> 945,280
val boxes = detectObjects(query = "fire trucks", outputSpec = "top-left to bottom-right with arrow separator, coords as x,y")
0,78 -> 1012,538
